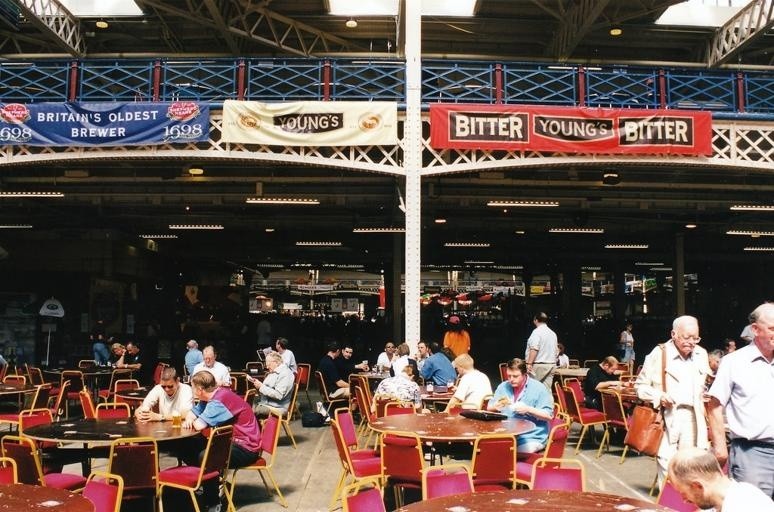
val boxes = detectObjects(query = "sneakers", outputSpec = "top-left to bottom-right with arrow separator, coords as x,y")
209,502 -> 222,512
218,482 -> 230,500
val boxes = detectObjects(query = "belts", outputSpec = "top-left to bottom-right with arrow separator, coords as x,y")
731,437 -> 774,449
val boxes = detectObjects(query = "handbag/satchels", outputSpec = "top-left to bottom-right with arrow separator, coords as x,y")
618,349 -> 626,358
302,410 -> 325,427
625,404 -> 664,457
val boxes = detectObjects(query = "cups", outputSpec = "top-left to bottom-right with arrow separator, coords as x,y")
182,375 -> 188,383
446,378 -> 454,393
376,364 -> 383,375
362,360 -> 367,370
371,366 -> 377,375
426,382 -> 433,396
171,409 -> 180,428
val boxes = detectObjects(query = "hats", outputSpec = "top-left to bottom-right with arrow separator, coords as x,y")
448,316 -> 460,324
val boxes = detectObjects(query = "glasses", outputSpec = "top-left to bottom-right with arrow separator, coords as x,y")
385,346 -> 395,349
680,336 -> 701,343
162,385 -> 173,389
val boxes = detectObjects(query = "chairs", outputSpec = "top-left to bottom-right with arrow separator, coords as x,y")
0,360 -> 701,512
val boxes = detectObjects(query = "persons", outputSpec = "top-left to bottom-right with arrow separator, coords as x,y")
0,356 -> 9,384
92,303 -> 774,512
